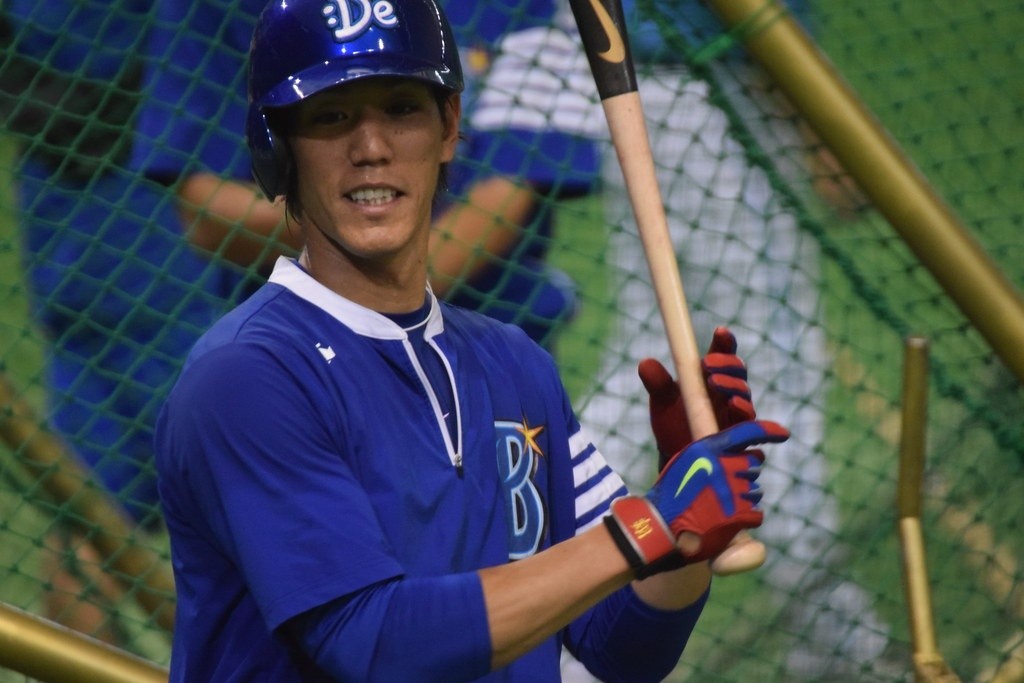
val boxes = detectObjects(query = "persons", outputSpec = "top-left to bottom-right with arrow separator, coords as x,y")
157,0 -> 793,683
133,2 -> 601,343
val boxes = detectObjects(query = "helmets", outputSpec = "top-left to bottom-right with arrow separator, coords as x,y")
243,0 -> 464,206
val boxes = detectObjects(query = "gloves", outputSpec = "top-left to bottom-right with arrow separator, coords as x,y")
603,421 -> 791,581
637,328 -> 755,471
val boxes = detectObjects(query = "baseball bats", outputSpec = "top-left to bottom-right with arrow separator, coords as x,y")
557,0 -> 770,580
